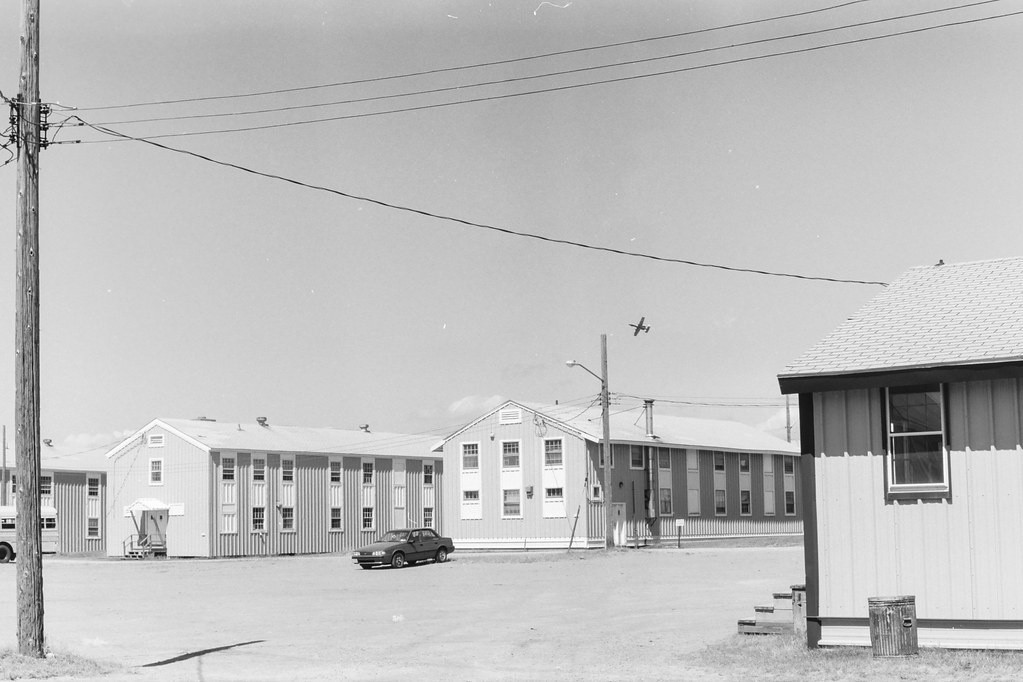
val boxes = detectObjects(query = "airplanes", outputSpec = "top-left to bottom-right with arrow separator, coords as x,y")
628,317 -> 651,337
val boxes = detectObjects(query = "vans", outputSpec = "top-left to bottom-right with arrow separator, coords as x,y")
0,505 -> 58,564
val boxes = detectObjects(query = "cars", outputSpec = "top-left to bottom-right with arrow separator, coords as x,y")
351,527 -> 454,569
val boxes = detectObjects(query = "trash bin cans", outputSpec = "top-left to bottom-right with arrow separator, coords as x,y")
867,594 -> 918,661
790,583 -> 808,637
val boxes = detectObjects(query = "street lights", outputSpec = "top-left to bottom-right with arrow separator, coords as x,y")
564,359 -> 615,547
